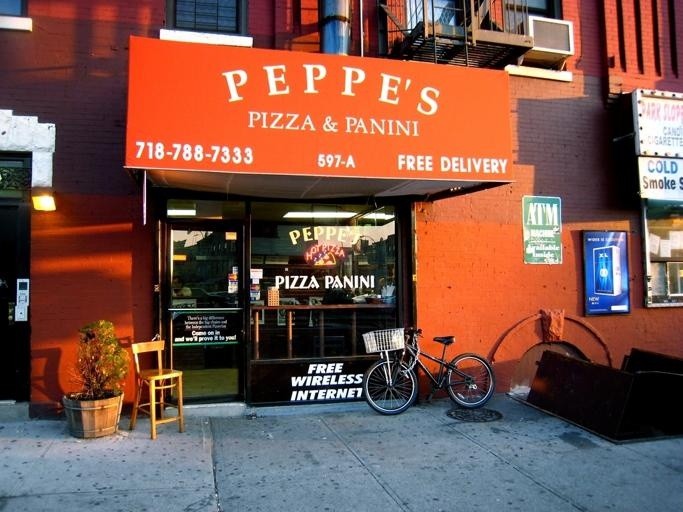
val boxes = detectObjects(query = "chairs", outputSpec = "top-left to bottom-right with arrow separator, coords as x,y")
125,338 -> 188,441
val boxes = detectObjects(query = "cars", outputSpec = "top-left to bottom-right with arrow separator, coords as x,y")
169,284 -> 240,310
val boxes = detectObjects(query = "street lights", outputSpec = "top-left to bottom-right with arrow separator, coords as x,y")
358,234 -> 376,245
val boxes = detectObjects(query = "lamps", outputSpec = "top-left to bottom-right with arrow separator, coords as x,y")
26,183 -> 58,214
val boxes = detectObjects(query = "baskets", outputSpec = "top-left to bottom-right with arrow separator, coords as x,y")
362,327 -> 406,355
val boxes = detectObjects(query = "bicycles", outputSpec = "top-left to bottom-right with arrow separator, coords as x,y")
361,326 -> 495,417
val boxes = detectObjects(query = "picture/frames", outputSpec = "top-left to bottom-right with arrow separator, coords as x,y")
577,227 -> 633,318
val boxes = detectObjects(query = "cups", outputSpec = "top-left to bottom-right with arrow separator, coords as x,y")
381,296 -> 393,304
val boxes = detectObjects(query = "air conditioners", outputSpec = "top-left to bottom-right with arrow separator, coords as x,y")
514,13 -> 574,71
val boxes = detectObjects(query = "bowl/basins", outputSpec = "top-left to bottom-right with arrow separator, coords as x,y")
365,297 -> 381,305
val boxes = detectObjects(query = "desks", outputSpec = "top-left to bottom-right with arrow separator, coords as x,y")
249,299 -> 398,360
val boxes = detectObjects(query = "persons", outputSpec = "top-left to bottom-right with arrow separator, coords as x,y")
172,276 -> 193,298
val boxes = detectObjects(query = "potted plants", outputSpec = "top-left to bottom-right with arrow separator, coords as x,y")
57,318 -> 131,442
